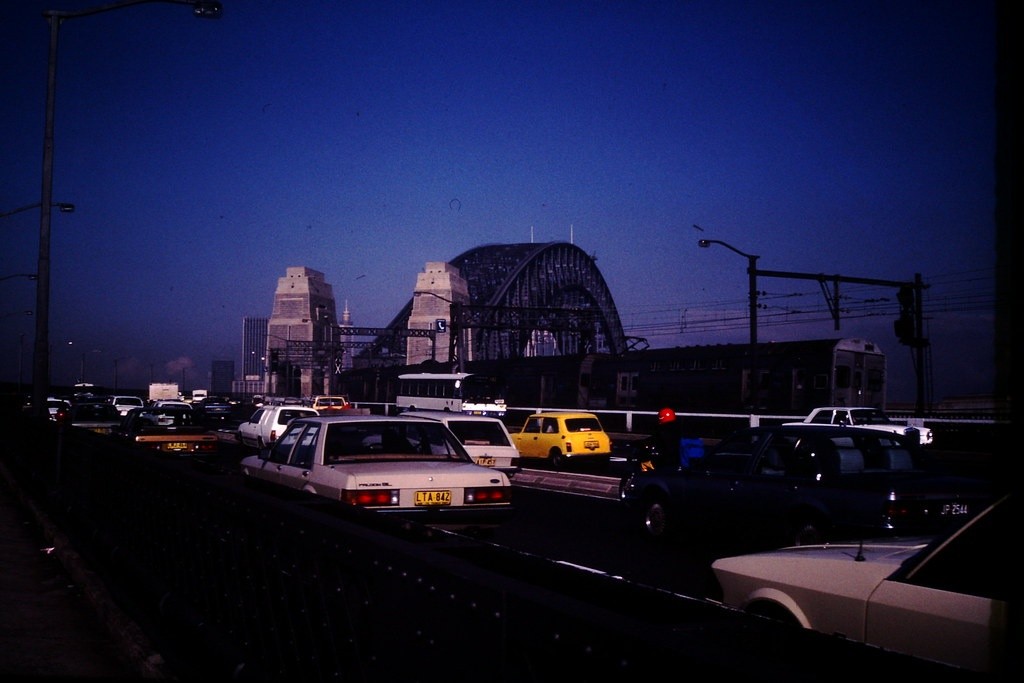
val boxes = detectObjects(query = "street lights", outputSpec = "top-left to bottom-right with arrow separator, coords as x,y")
698,240 -> 761,360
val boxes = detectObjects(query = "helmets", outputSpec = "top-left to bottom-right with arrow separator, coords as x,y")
655,407 -> 676,425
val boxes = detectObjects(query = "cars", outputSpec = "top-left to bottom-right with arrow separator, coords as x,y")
44,389 -> 371,463
617,422 -> 975,559
782,406 -> 934,447
239,404 -> 515,527
501,411 -> 613,471
361,410 -> 522,481
710,482 -> 1024,682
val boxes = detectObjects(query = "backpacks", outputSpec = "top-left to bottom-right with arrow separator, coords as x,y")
677,434 -> 708,470
